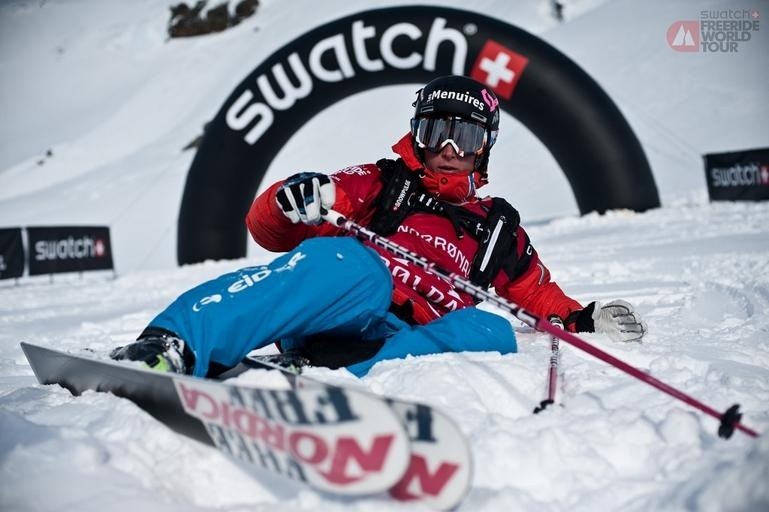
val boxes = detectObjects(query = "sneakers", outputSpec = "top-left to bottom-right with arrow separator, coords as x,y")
249,353 -> 313,375
110,334 -> 188,372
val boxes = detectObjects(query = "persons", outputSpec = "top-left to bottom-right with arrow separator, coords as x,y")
104,74 -> 649,383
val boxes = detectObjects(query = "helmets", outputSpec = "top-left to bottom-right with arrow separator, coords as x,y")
412,76 -> 500,165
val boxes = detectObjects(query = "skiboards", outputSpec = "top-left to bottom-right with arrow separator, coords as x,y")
19,341 -> 474,512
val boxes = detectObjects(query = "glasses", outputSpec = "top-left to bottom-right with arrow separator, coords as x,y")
414,118 -> 489,157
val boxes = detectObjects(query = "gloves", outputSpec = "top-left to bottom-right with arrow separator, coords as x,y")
576,299 -> 645,341
274,172 -> 338,226
